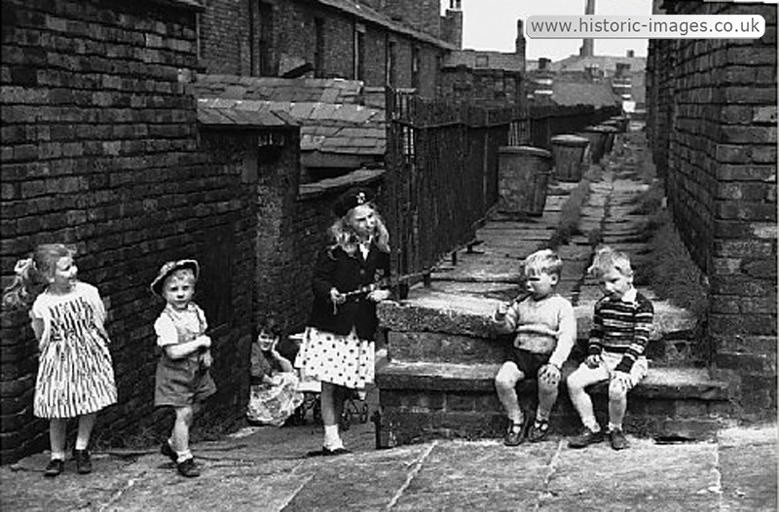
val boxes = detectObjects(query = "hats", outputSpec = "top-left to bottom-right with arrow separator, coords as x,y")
151,259 -> 201,298
332,186 -> 376,218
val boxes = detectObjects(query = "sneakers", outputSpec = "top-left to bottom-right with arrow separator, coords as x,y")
160,440 -> 176,458
607,427 -> 631,449
567,427 -> 604,447
527,417 -> 549,443
72,448 -> 93,475
506,416 -> 529,447
45,457 -> 64,479
320,445 -> 354,458
175,455 -> 200,478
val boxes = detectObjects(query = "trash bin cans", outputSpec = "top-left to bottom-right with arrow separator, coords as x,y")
496,146 -> 552,216
572,115 -> 631,165
550,134 -> 590,182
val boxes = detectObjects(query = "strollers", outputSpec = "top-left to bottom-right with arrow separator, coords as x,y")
280,332 -> 371,430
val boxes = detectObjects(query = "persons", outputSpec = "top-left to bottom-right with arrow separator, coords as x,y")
562,244 -> 653,449
492,249 -> 579,446
293,187 -> 393,453
147,256 -> 219,477
3,241 -> 117,477
246,317 -> 303,428
